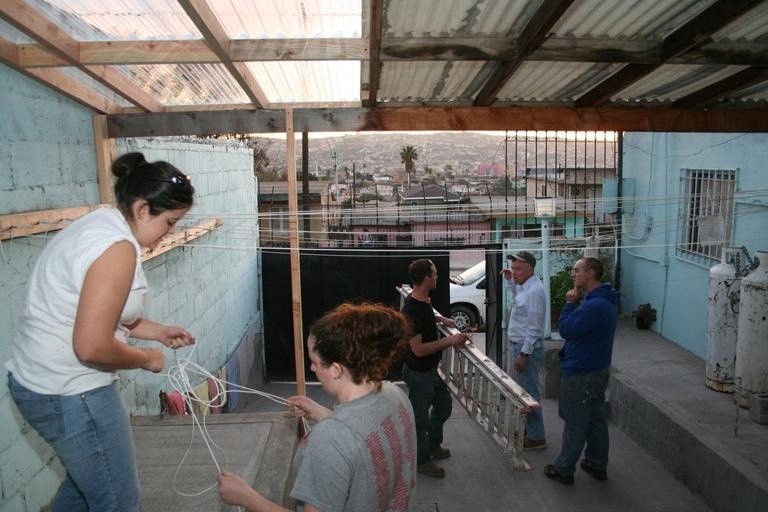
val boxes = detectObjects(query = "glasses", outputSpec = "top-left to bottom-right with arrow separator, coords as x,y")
148,175 -> 192,185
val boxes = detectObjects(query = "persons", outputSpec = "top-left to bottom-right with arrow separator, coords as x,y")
499,251 -> 547,451
399,258 -> 475,480
6,151 -> 198,511
217,302 -> 417,510
543,258 -> 619,484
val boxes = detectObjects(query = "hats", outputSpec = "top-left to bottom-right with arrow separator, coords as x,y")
506,251 -> 537,268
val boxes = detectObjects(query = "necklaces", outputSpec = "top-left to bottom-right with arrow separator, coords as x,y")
411,290 -> 430,304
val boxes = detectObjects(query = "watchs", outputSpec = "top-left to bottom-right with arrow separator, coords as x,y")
519,352 -> 530,358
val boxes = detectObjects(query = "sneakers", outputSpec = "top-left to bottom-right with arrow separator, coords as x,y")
417,462 -> 445,478
581,458 -> 608,481
544,464 -> 575,485
512,429 -> 526,438
430,446 -> 450,458
523,438 -> 546,450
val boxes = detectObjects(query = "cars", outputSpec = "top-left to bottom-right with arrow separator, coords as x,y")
449,259 -> 486,332
